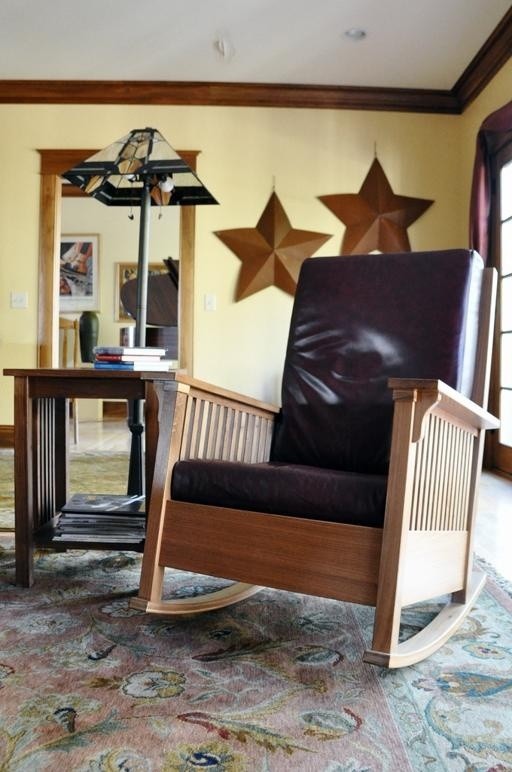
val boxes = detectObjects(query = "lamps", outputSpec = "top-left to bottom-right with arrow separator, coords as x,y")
52,128 -> 218,507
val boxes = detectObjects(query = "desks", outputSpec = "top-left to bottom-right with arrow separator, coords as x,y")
0,358 -> 165,596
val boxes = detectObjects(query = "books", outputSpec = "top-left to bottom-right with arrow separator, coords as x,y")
92,347 -> 169,371
52,494 -> 147,544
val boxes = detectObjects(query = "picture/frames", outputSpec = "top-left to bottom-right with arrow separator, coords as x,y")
111,256 -> 170,326
58,230 -> 101,320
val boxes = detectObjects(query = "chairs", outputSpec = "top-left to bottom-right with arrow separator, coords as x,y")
58,316 -> 80,447
128,241 -> 503,677
